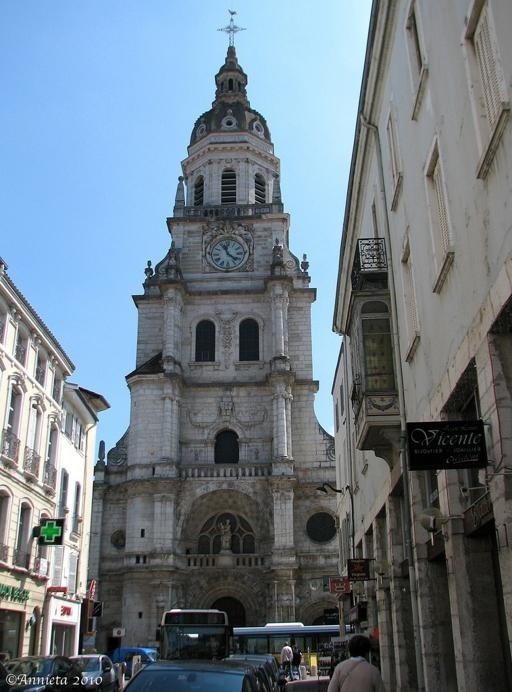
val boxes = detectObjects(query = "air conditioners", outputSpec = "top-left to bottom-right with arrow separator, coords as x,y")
94,601 -> 103,618
88,617 -> 96,632
112,628 -> 125,637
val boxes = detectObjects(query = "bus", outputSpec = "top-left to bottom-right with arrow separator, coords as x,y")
230,621 -> 355,674
157,607 -> 237,661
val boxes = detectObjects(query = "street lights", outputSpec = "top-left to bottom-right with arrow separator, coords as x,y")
315,482 -> 358,605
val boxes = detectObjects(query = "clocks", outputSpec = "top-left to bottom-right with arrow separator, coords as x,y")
211,239 -> 245,269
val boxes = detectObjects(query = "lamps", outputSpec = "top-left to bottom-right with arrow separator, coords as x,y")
315,483 -> 344,495
26,617 -> 36,632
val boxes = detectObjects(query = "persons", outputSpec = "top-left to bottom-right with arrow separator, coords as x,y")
328,636 -> 389,692
281,641 -> 303,681
220,520 -> 232,550
